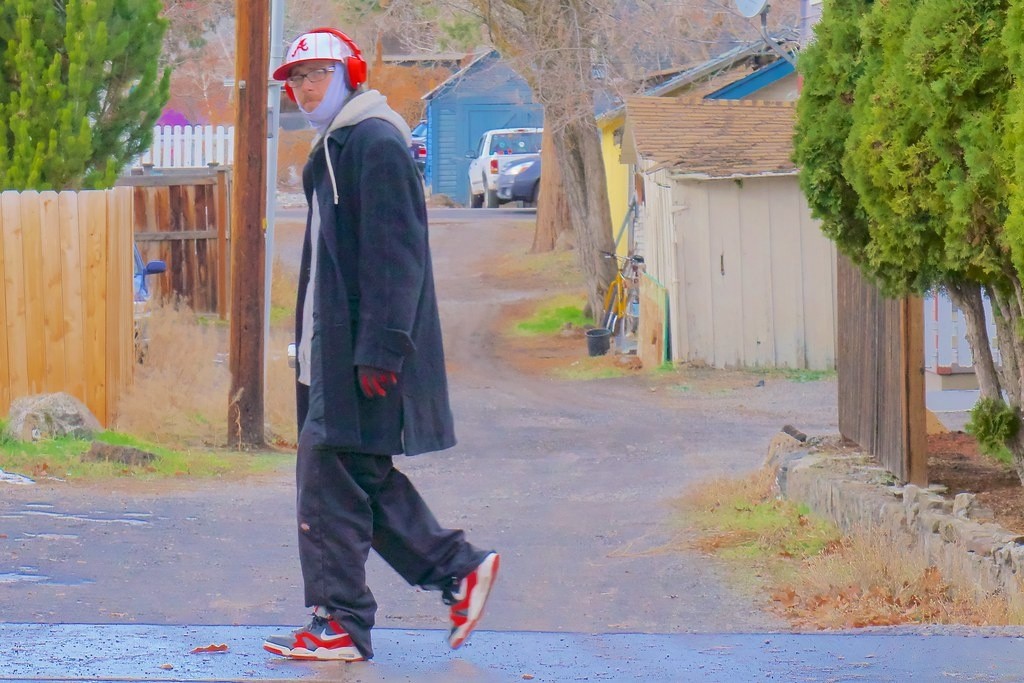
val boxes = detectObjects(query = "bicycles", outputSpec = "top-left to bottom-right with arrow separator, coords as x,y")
599,250 -> 645,339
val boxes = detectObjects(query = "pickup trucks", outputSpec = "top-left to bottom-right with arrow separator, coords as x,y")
465,127 -> 545,208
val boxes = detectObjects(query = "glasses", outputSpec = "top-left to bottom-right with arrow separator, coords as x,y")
285,65 -> 336,88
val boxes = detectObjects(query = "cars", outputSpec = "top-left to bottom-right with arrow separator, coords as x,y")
496,157 -> 545,207
409,120 -> 428,178
134,243 -> 166,349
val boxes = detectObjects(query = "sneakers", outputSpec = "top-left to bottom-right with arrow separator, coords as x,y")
442,553 -> 499,650
262,605 -> 364,661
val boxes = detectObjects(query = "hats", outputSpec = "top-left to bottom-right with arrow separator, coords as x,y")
273,31 -> 353,81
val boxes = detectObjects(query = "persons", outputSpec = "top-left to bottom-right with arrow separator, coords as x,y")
262,26 -> 499,662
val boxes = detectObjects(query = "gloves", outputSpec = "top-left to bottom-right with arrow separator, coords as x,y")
357,365 -> 398,400
